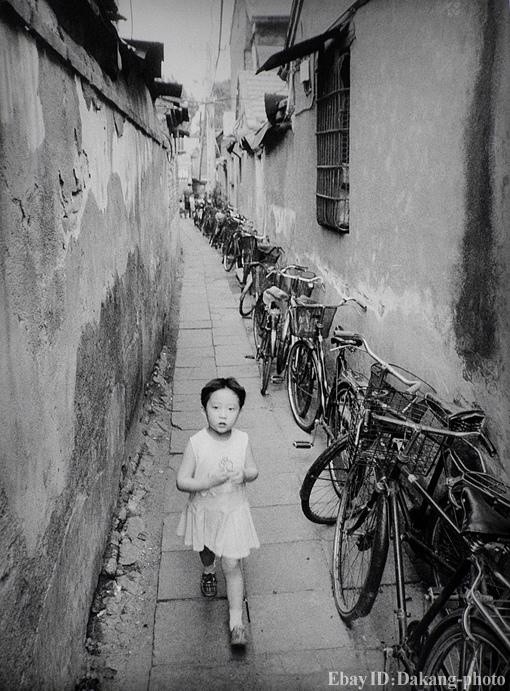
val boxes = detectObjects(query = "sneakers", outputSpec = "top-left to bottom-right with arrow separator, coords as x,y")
230,626 -> 248,652
200,572 -> 218,598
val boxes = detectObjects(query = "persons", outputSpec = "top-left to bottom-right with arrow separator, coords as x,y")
179,200 -> 184,218
189,193 -> 195,218
183,184 -> 193,218
174,378 -> 261,650
